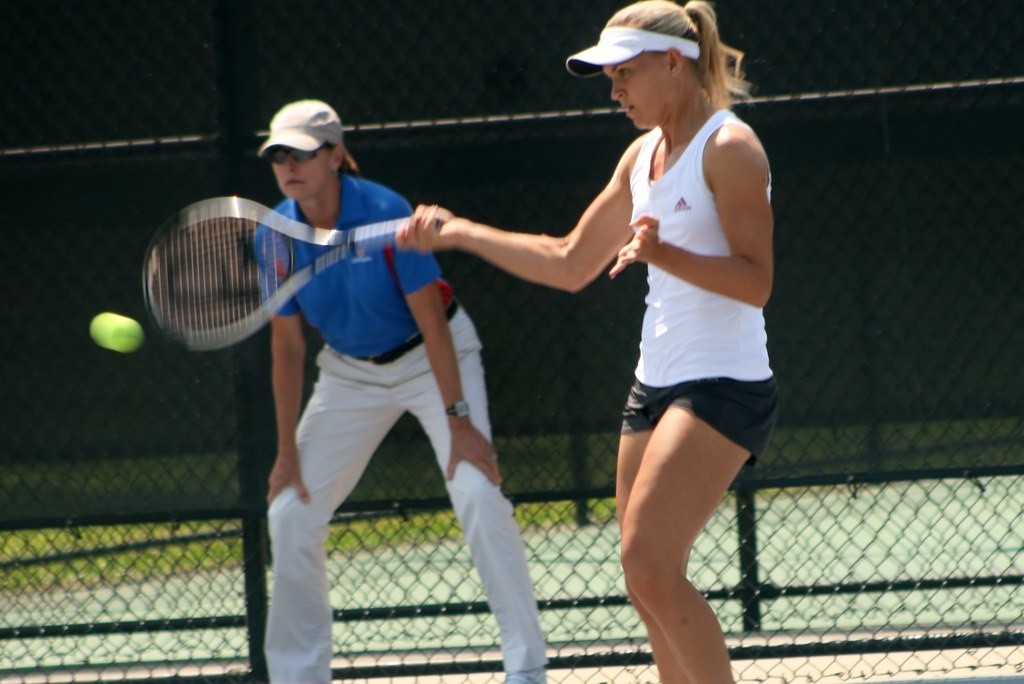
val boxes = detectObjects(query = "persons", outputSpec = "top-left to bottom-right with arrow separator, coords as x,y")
250,98 -> 549,684
393,0 -> 777,684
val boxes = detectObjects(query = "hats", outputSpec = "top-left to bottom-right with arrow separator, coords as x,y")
565,25 -> 701,79
256,100 -> 344,158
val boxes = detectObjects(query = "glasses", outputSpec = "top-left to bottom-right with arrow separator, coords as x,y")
268,146 -> 319,164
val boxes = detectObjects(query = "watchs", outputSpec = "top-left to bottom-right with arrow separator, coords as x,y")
445,399 -> 470,417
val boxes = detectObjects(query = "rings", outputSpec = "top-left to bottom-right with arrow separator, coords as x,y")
491,453 -> 498,460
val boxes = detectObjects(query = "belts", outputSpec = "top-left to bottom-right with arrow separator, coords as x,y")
374,298 -> 463,367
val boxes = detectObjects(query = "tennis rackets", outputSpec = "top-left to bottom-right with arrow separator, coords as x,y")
141,195 -> 443,352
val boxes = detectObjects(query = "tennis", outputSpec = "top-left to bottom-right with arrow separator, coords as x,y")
88,311 -> 144,354
